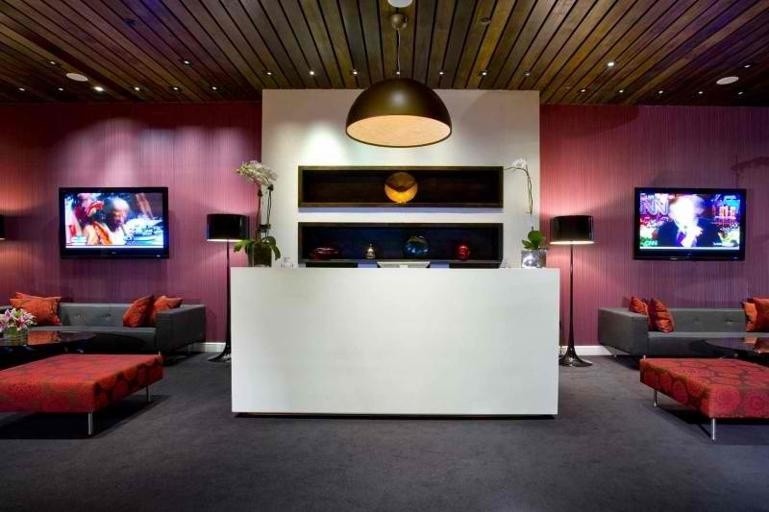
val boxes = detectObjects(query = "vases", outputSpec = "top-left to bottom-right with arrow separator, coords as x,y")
248,242 -> 272,268
4,329 -> 27,339
521,250 -> 547,268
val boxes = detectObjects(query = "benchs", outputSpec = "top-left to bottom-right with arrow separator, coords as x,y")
640,352 -> 768,440
0,353 -> 164,438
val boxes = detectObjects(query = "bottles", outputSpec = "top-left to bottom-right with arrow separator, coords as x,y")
403,234 -> 432,258
365,244 -> 375,259
458,245 -> 471,260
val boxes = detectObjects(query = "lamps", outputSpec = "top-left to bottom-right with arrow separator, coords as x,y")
551,215 -> 594,368
206,212 -> 249,362
346,13 -> 453,149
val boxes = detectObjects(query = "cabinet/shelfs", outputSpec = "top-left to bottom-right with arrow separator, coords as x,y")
292,162 -> 505,272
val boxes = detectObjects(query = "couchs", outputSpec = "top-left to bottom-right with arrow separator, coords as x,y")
1,298 -> 205,362
598,300 -> 769,360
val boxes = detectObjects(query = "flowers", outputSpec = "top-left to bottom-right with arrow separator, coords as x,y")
233,159 -> 282,266
507,155 -> 549,247
0,308 -> 37,332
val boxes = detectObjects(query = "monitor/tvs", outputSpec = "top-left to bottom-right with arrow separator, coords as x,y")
449,263 -> 501,268
377,260 -> 431,268
306,262 -> 358,267
634,188 -> 746,261
59,186 -> 169,259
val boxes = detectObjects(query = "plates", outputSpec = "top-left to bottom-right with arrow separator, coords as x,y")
385,172 -> 418,202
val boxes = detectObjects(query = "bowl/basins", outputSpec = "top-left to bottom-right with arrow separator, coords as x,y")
313,248 -> 340,260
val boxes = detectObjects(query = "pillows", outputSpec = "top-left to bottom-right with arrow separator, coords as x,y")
122,294 -> 184,329
742,294 -> 769,332
8,291 -> 61,326
631,294 -> 672,333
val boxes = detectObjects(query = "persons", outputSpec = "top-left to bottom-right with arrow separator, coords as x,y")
63,192 -> 136,246
653,193 -> 720,246
